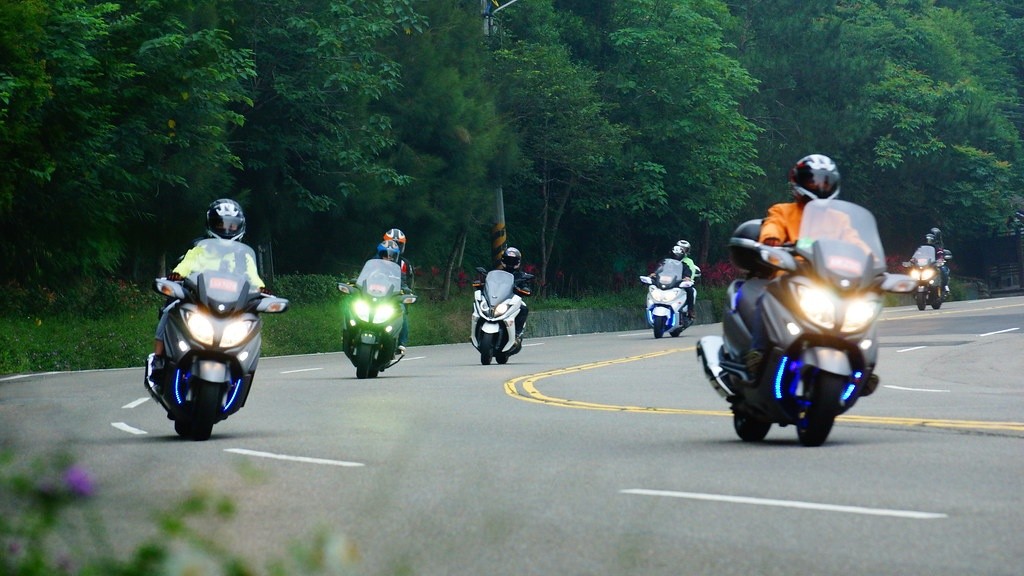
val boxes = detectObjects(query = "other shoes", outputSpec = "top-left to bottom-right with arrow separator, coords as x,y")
857,374 -> 878,396
688,310 -> 695,318
742,347 -> 764,380
945,285 -> 950,293
514,336 -> 519,346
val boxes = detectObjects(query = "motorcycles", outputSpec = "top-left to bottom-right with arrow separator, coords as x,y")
337,259 -> 419,380
639,258 -> 698,339
144,238 -> 288,442
902,246 -> 953,311
697,198 -> 918,446
471,266 -> 535,366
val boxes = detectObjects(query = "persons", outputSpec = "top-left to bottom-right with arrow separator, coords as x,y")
909,228 -> 950,297
152,198 -> 271,379
473,247 -> 532,346
741,154 -> 879,397
649,240 -> 701,321
348,229 -> 415,355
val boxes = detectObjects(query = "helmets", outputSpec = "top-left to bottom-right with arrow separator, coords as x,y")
376,240 -> 400,263
500,247 -> 522,269
382,228 -> 406,254
669,246 -> 685,261
922,234 -> 936,245
787,154 -> 841,203
931,227 -> 942,240
204,198 -> 247,247
675,239 -> 691,257
